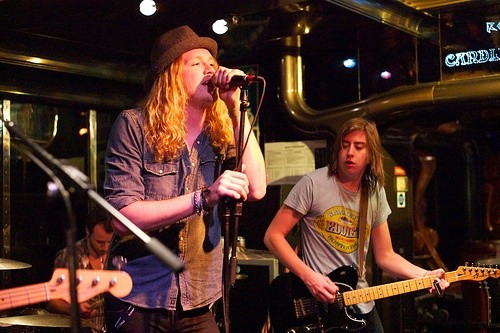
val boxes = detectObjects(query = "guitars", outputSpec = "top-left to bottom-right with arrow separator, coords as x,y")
264,259 -> 499,332
0,266 -> 137,313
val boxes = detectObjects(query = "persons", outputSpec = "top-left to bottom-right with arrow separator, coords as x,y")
48,209 -> 114,319
101,25 -> 267,333
263,119 -> 449,333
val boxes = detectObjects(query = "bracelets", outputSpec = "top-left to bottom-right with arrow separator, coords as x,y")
193,188 -> 212,216
422,271 -> 430,276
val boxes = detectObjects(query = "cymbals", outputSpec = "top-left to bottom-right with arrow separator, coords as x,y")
0,312 -> 95,330
222,246 -> 276,261
0,258 -> 33,271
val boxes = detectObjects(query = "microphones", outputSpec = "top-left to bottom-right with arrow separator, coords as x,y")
229,75 -> 260,87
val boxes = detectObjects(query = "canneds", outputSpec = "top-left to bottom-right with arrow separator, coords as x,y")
236,236 -> 245,253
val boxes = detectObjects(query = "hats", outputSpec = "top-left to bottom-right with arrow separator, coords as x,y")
145,25 -> 217,95
